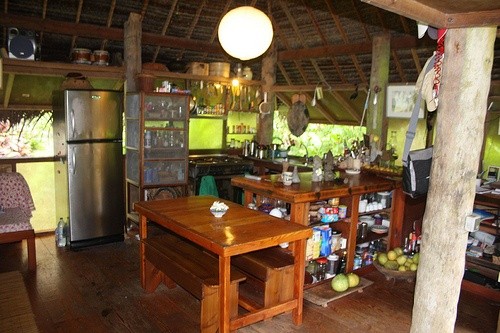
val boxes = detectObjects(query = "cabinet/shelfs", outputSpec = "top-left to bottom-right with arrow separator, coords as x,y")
232,168 -> 408,289
125,91 -> 191,242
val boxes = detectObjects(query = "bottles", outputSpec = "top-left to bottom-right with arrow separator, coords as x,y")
330,231 -> 342,251
377,192 -> 391,209
326,254 -> 340,275
144,131 -> 175,148
364,255 -> 373,265
338,205 -> 347,218
56,217 -> 66,247
360,223 -> 368,239
198,102 -> 225,115
315,258 -> 328,281
243,67 -> 252,80
410,231 -> 417,252
230,138 -> 241,149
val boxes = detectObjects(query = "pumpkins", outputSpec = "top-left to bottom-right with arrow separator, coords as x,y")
332,272 -> 360,292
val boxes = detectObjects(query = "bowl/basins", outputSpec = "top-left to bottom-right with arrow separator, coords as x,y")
210,209 -> 227,218
270,143 -> 279,150
361,218 -> 389,235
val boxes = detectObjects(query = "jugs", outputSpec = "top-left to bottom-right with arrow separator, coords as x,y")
244,140 -> 267,160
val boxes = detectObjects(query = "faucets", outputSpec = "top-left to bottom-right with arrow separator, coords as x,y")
300,143 -> 309,166
286,144 -> 295,163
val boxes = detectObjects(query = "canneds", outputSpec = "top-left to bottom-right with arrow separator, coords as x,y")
338,205 -> 347,218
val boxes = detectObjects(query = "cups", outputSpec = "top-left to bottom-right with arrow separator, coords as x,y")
359,199 -> 383,212
282,172 -> 293,186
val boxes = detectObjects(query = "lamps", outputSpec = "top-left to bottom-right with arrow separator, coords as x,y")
217,0 -> 274,60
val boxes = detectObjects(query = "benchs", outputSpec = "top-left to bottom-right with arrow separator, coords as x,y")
233,245 -> 296,321
0,270 -> 40,333
142,233 -> 247,333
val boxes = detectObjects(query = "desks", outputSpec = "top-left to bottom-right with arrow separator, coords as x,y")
0,209 -> 36,292
132,195 -> 314,333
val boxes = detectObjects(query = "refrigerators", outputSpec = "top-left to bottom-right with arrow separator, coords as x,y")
52,89 -> 125,248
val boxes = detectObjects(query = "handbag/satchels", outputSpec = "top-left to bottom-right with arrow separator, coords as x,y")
402,146 -> 433,198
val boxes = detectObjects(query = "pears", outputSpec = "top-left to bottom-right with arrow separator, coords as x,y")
378,247 -> 419,271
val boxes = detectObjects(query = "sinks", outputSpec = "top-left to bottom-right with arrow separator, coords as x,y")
275,158 -> 302,164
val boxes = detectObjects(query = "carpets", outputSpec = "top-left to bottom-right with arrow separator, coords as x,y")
304,272 -> 373,306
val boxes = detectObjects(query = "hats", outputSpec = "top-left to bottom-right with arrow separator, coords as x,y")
415,51 -> 444,112
